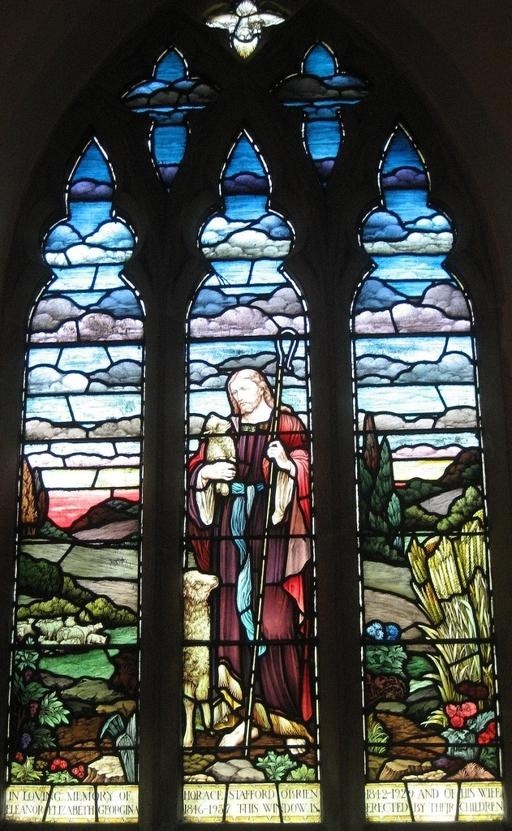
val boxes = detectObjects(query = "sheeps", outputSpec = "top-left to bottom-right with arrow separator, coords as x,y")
198,412 -> 237,496
181,568 -> 220,746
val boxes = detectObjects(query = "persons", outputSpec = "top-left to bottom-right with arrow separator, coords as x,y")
188,369 -> 315,757
